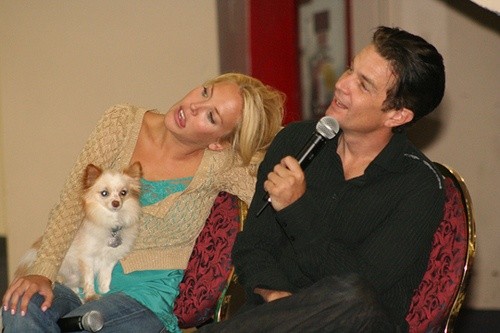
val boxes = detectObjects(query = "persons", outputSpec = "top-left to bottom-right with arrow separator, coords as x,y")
0,73 -> 285,333
191,26 -> 446,333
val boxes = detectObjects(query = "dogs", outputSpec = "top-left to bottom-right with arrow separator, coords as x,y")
57,161 -> 145,305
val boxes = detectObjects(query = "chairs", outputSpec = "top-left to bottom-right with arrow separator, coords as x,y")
173,190 -> 247,333
406,162 -> 477,333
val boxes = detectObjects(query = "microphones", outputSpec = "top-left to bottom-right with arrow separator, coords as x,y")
55,310 -> 104,333
256,116 -> 340,217
